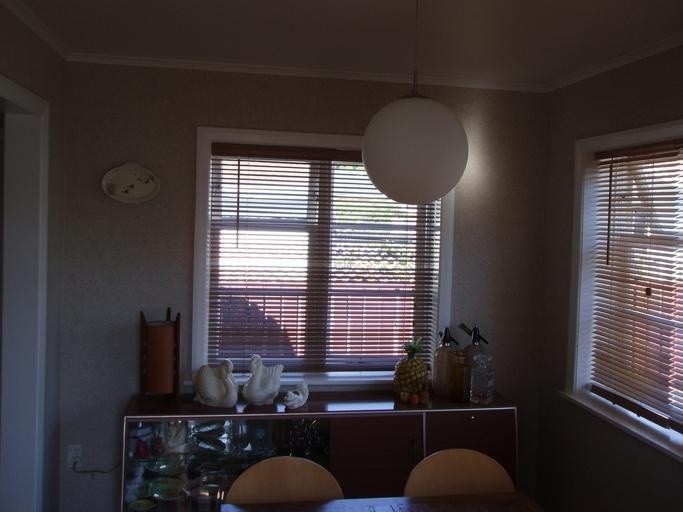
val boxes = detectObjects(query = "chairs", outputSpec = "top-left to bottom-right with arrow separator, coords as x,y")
401,446 -> 516,497
223,454 -> 346,503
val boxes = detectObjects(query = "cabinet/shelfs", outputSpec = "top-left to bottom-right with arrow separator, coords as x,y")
118,389 -> 520,512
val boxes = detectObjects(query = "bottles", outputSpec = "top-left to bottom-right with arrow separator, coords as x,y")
462,324 -> 485,363
432,323 -> 456,396
446,351 -> 470,403
470,354 -> 494,403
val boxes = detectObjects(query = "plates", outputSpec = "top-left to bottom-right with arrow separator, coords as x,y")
128,422 -> 231,512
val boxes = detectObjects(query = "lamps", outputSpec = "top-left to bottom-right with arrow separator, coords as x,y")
359,0 -> 470,209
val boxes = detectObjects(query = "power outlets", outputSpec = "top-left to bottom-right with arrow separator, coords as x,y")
66,443 -> 82,471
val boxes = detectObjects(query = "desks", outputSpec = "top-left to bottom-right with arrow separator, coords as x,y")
219,493 -> 545,512
